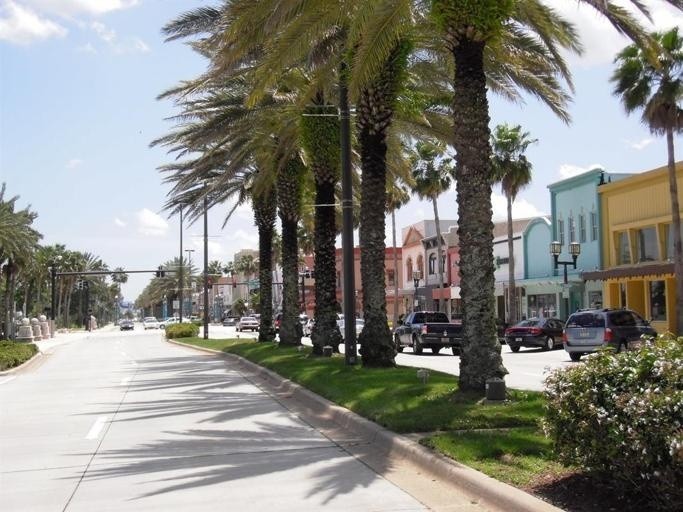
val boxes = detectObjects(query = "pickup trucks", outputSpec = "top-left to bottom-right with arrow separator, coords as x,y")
393,310 -> 463,356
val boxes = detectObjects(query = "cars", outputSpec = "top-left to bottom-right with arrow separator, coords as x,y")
235,316 -> 260,332
222,318 -> 239,326
118,315 -> 201,331
504,316 -> 566,353
271,312 -> 365,344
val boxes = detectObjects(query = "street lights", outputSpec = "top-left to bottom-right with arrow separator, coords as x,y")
411,269 -> 423,312
172,198 -> 196,328
548,240 -> 581,322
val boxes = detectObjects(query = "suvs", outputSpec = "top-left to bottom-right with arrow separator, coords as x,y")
562,304 -> 659,363
